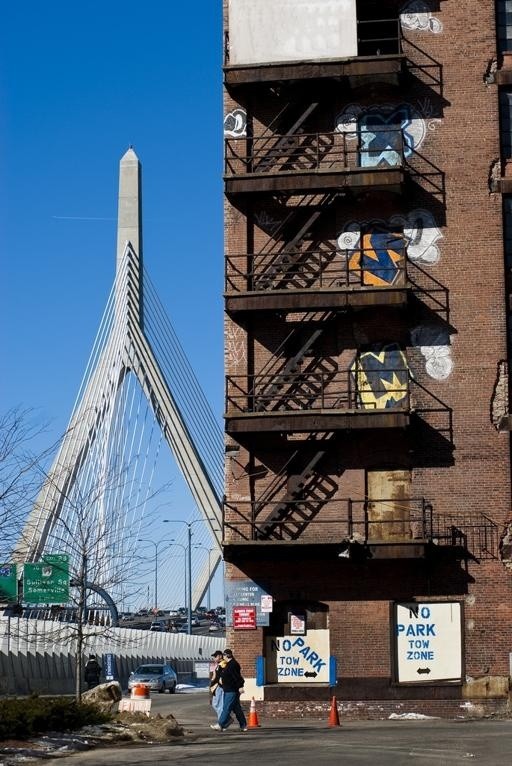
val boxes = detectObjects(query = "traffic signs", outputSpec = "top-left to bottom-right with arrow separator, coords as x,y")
42,555 -> 69,562
0,563 -> 19,604
24,563 -> 71,604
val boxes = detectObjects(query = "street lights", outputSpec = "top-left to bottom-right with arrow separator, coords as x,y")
137,539 -> 178,624
168,542 -> 202,617
163,517 -> 216,636
194,546 -> 224,612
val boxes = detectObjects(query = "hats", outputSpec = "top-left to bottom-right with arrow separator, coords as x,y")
211,650 -> 223,657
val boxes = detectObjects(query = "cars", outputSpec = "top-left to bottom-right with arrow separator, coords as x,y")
126,663 -> 180,696
121,606 -> 228,637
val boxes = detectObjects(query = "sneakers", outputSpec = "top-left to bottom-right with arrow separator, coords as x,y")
209,723 -> 223,732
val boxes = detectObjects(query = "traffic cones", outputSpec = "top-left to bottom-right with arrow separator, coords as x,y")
246,698 -> 262,729
327,695 -> 344,729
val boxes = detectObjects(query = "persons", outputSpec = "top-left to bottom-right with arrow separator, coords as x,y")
85,656 -> 103,689
210,649 -> 249,732
213,651 -> 233,730
